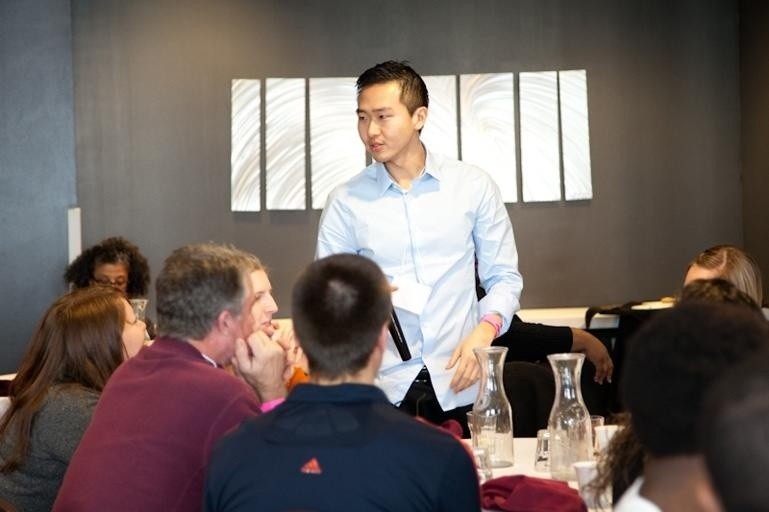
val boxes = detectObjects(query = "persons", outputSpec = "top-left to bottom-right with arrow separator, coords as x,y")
311,58 -> 524,443
2,237 -> 306,511
203,254 -> 482,511
590,244 -> 767,510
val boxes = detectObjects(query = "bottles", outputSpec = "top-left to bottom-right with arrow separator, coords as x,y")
545,352 -> 596,481
469,344 -> 516,469
128,298 -> 149,341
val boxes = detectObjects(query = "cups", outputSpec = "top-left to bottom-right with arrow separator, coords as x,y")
459,408 -> 632,511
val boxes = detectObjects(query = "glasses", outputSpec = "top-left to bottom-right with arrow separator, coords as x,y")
92,276 -> 130,289
